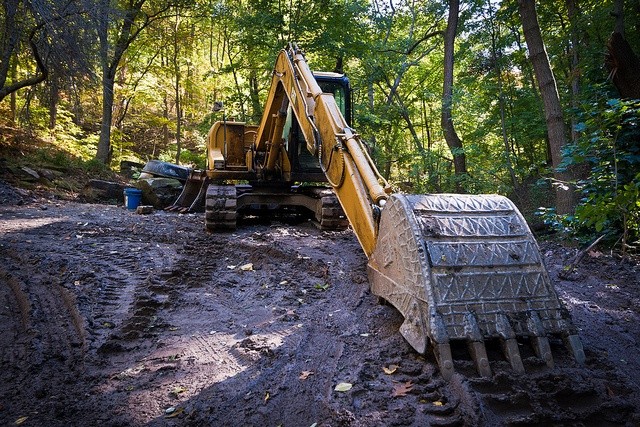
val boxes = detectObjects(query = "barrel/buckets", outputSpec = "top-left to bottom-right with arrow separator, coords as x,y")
123,188 -> 142,209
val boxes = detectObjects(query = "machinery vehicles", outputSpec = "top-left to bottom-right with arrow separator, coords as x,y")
164,42 -> 586,380
